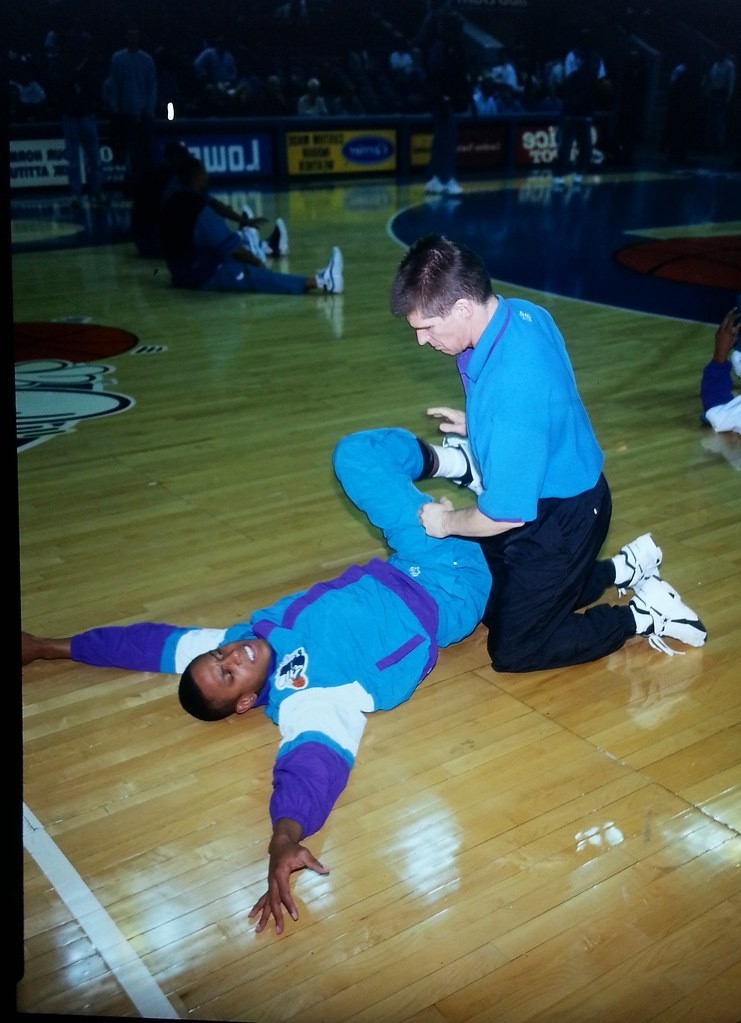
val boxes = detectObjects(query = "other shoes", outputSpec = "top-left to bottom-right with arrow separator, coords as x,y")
424,176 -> 442,194
446,178 -> 463,195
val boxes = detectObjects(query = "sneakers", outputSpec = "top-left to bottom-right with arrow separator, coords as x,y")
442,437 -> 482,497
628,574 -> 709,657
616,532 -> 663,599
265,218 -> 289,259
238,204 -> 254,232
318,246 -> 344,294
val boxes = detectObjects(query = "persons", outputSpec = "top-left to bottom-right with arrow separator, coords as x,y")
710,54 -> 736,152
551,33 -> 607,186
8,1 -> 430,118
700,305 -> 741,434
21,427 -> 493,934
132,144 -> 344,295
426,49 -> 467,197
390,234 -> 707,674
412,0 -> 713,116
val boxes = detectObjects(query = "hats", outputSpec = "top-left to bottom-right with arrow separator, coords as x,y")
307,78 -> 320,88
